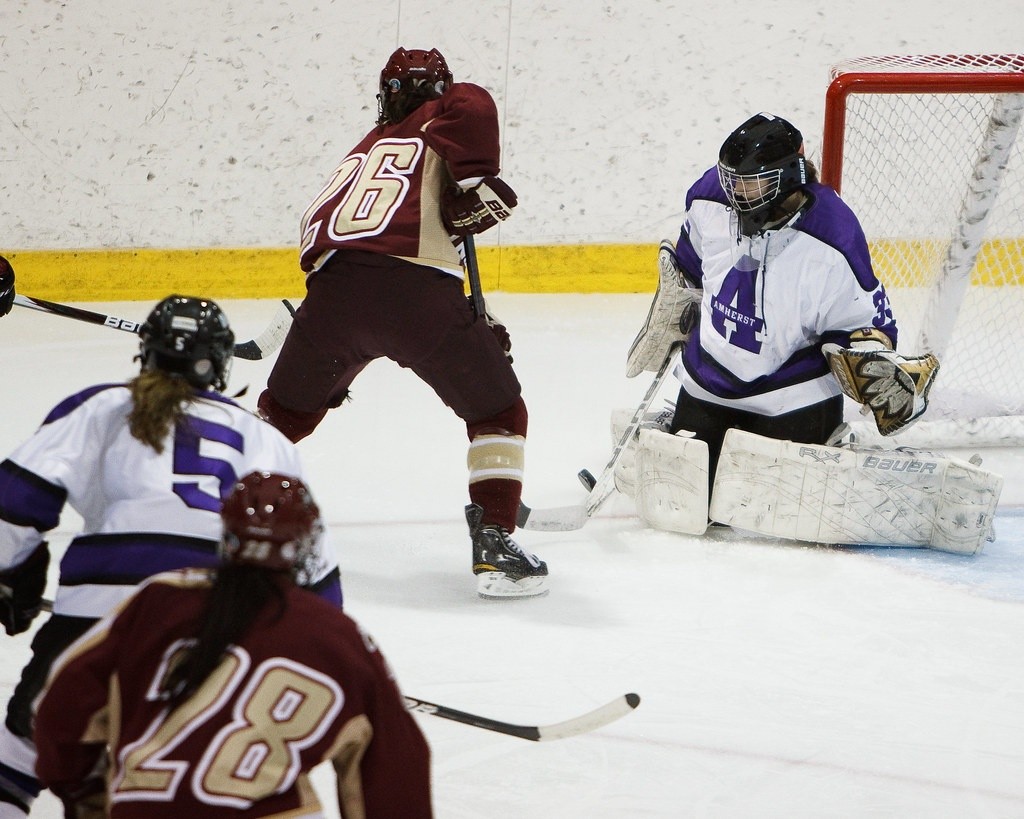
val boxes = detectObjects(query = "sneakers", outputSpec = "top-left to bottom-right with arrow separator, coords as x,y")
465,503 -> 550,598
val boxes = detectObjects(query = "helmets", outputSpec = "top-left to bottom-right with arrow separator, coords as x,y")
719,111 -> 806,219
139,293 -> 236,390
217,470 -> 323,568
379,46 -> 454,95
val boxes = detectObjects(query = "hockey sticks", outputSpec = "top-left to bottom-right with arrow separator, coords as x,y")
12,293 -> 295,361
402,692 -> 642,744
514,342 -> 683,534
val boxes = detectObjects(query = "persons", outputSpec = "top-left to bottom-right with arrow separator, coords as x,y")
29,470 -> 433,818
1,293 -> 344,819
624,110 -> 939,530
246,46 -> 555,600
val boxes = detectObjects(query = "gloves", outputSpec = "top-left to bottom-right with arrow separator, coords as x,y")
467,294 -> 514,364
440,174 -> 520,235
0,540 -> 51,637
